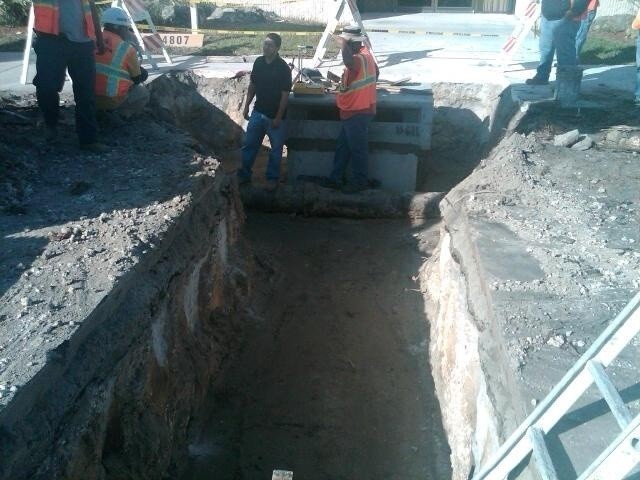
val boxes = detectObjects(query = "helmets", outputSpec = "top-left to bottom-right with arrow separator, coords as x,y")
100,7 -> 131,26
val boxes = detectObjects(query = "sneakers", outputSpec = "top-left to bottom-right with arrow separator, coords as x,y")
525,77 -> 549,85
342,181 -> 365,193
81,142 -> 109,152
321,178 -> 347,187
45,126 -> 58,144
264,177 -> 279,192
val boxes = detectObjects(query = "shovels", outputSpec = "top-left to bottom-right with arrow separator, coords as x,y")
376,79 -> 410,86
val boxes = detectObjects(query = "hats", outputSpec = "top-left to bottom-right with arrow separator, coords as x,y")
334,26 -> 366,43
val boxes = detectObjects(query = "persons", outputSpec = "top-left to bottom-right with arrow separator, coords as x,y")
525,1 -> 589,87
31,1 -> 112,154
629,9 -> 639,105
577,0 -> 600,68
232,33 -> 292,194
93,6 -> 152,122
329,22 -> 380,194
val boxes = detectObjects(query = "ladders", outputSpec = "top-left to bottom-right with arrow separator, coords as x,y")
469,290 -> 640,480
110,0 -> 172,70
311,0 -> 379,79
